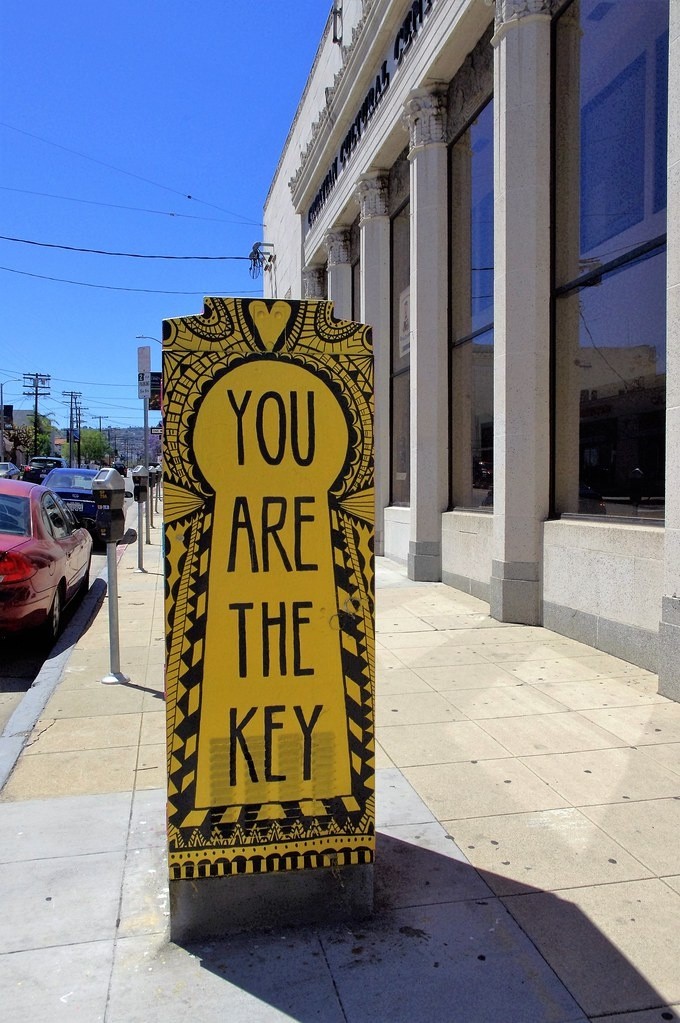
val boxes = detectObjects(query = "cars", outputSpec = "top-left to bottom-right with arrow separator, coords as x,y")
35,467 -> 98,527
111,462 -> 128,477
22,456 -> 70,484
0,461 -> 20,479
0,478 -> 94,640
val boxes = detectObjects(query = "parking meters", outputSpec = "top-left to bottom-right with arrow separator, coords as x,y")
91,463 -> 164,685
